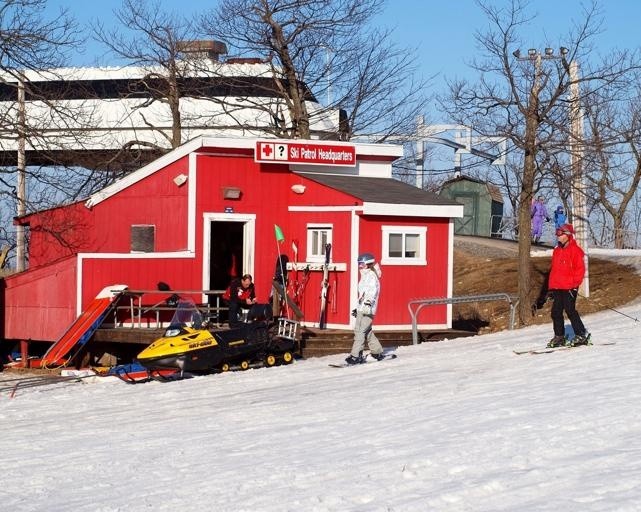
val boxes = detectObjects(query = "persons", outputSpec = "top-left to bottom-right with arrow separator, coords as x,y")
222,273 -> 257,306
345,253 -> 384,364
547,224 -> 590,346
531,196 -> 550,242
553,205 -> 567,228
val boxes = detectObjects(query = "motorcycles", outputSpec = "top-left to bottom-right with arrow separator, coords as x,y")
109,300 -> 303,384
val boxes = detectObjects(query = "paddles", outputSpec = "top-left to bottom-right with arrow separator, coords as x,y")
157,282 -> 207,314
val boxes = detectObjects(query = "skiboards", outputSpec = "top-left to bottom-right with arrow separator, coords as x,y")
328,354 -> 396,367
319,243 -> 332,330
512,340 -> 616,354
273,279 -> 304,317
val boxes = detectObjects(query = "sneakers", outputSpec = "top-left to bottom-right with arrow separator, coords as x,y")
345,356 -> 364,364
547,336 -> 566,347
366,352 -> 382,361
570,331 -> 591,346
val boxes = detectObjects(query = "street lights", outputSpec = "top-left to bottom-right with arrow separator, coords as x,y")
513,44 -> 590,301
315,40 -> 332,112
1,67 -> 28,276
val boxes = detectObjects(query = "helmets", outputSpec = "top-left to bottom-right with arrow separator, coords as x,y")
357,253 -> 376,265
556,224 -> 575,235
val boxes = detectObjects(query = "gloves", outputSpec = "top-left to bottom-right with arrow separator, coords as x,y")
567,289 -> 578,301
362,302 -> 373,316
